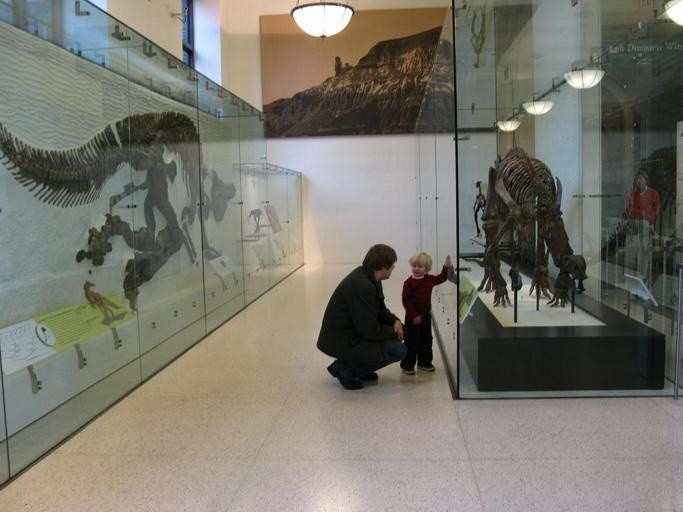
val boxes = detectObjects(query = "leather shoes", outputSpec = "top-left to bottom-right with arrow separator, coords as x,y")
364,373 -> 378,381
326,361 -> 364,389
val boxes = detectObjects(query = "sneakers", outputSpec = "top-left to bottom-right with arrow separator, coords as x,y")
417,364 -> 436,371
403,369 -> 414,374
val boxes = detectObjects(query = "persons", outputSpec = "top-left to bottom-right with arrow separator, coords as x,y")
449,256 -> 456,284
624,169 -> 661,279
316,244 -> 406,389
399,252 -> 450,374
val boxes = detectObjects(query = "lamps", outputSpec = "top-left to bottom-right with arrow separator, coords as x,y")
495,53 -> 608,136
287,0 -> 354,42
